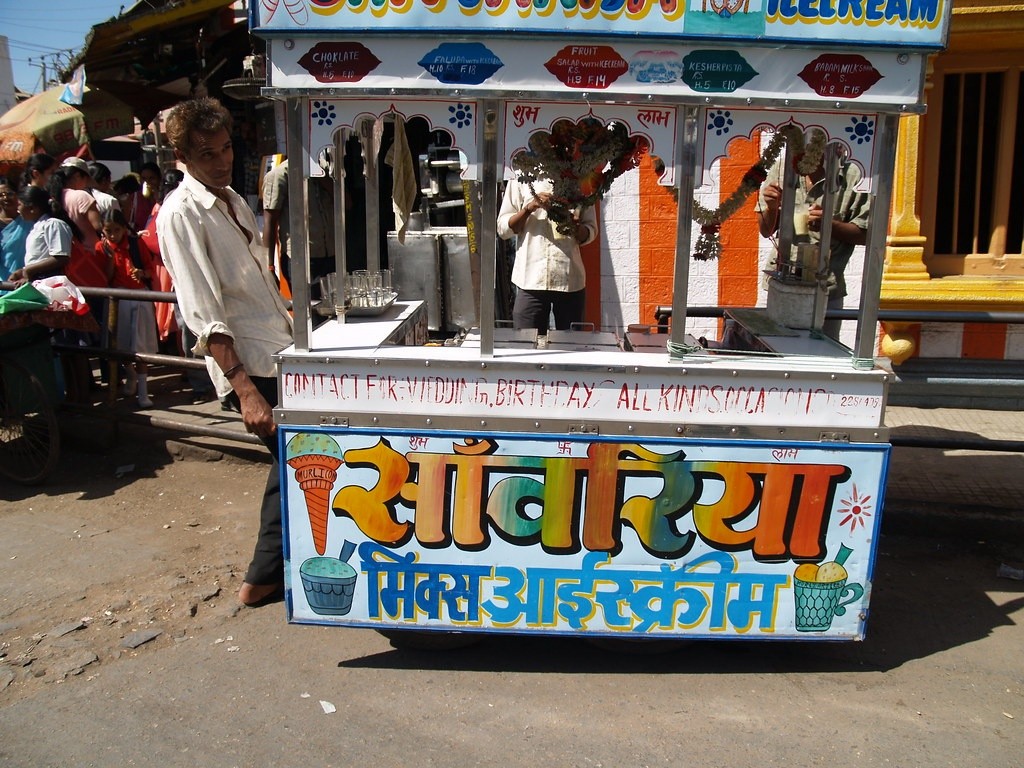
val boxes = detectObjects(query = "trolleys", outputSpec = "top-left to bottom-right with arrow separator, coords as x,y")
248,0 -> 955,644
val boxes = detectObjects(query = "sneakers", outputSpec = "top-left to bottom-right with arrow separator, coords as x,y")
138,395 -> 152,408
123,373 -> 138,396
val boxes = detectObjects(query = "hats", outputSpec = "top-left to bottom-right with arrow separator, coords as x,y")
60,156 -> 94,182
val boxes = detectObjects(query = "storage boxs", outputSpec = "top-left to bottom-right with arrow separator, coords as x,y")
768,280 -> 828,330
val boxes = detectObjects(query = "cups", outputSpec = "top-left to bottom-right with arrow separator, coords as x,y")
793,203 -> 810,235
319,268 -> 391,308
551,220 -> 565,240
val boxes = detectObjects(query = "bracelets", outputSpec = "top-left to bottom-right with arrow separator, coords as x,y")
222,362 -> 243,377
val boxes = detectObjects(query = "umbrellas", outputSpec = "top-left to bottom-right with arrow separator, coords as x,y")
0,70 -> 135,164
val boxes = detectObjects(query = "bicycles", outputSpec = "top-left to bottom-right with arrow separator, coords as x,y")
0,353 -> 61,488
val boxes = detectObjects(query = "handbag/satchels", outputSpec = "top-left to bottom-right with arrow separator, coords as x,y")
0,275 -> 90,316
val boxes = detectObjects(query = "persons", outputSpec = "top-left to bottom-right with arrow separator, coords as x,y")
754,148 -> 870,342
259,143 -> 344,331
1,153 -> 168,411
155,97 -> 299,606
495,164 -> 597,336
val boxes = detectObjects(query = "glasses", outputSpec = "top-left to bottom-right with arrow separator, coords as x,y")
0,191 -> 15,198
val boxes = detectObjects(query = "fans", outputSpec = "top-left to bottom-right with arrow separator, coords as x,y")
222,70 -> 270,101
